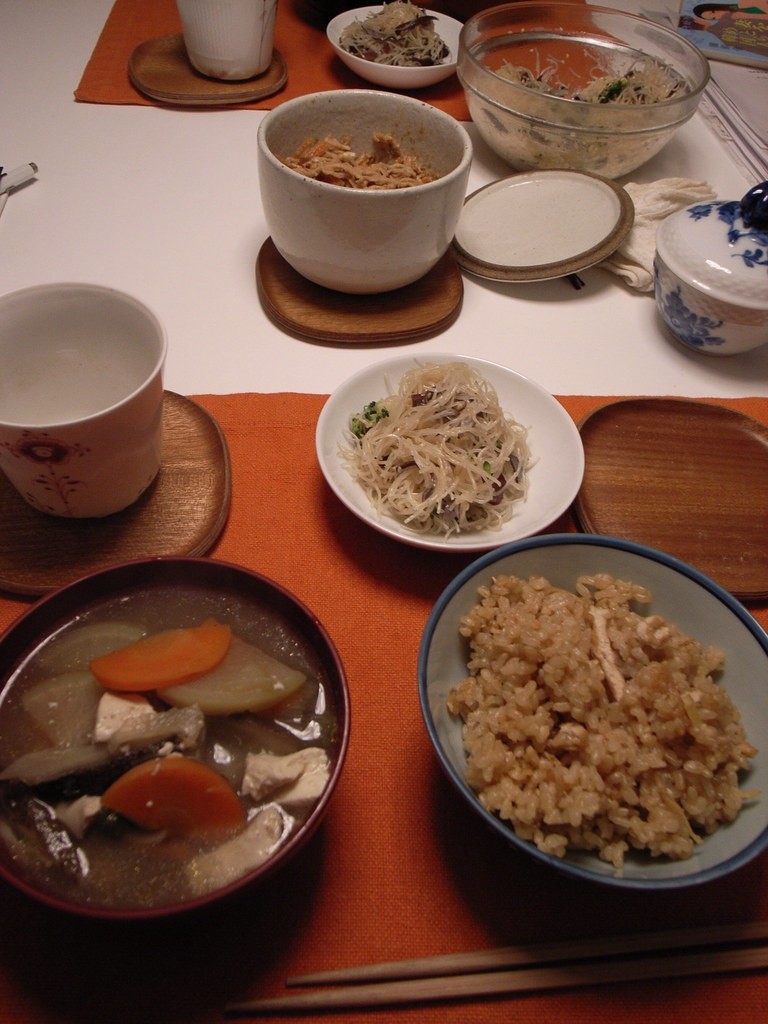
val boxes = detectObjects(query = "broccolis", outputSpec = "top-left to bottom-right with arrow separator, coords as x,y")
352,400 -> 391,434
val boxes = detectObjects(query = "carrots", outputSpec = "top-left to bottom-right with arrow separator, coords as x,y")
90,619 -> 232,693
99,756 -> 247,844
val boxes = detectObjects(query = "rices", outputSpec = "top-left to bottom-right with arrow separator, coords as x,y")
445,574 -> 765,867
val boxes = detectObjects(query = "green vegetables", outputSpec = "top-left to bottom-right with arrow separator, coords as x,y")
598,80 -> 623,103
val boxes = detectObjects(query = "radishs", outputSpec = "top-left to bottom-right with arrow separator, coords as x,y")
0,620 -> 309,792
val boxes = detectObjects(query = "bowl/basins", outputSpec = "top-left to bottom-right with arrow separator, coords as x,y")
455,2 -> 711,180
0,554 -> 352,921
417,533 -> 768,890
326,6 -> 464,89
315,351 -> 586,552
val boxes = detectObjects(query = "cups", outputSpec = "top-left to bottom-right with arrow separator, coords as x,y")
176,0 -> 277,81
256,89 -> 473,293
652,180 -> 768,355
0,280 -> 169,518
